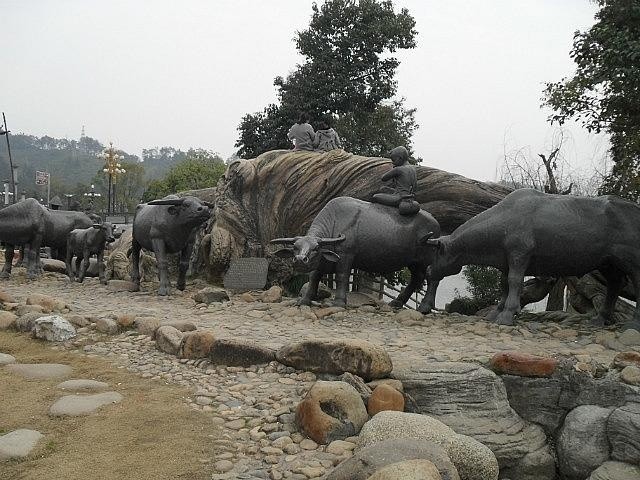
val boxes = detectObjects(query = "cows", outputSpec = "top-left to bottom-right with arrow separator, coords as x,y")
125,192 -> 219,294
0,196 -> 120,284
266,193 -> 444,316
418,185 -> 639,328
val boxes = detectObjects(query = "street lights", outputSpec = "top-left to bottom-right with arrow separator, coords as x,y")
85,141 -> 126,217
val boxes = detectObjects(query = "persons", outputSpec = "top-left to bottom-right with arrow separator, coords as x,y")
287,112 -> 316,152
372,146 -> 417,207
312,121 -> 342,152
